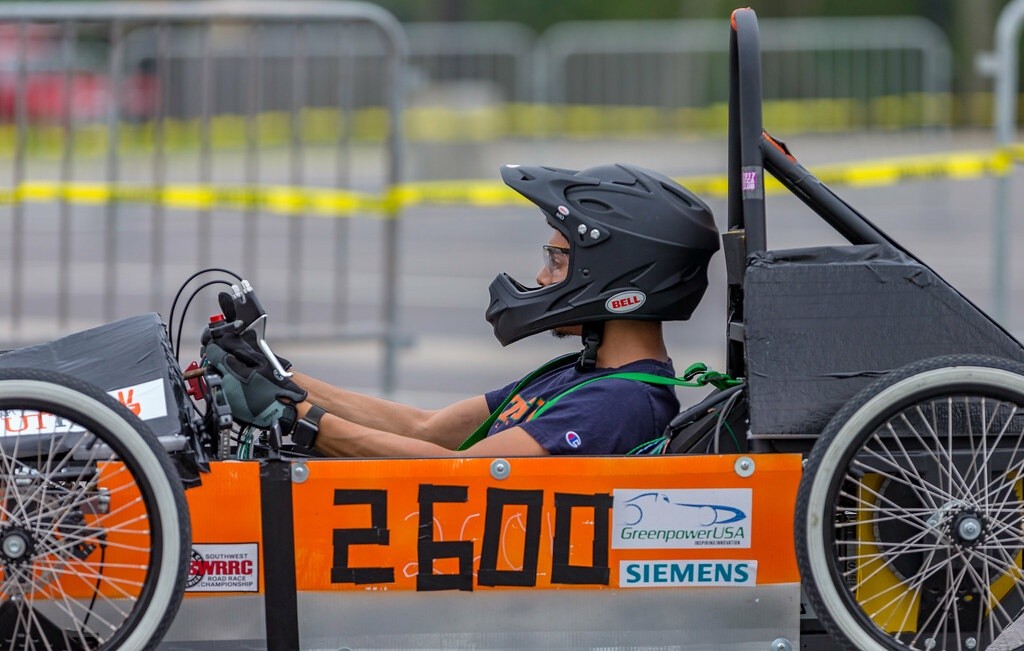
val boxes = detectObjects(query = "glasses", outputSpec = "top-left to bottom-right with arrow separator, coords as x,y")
542,245 -> 570,278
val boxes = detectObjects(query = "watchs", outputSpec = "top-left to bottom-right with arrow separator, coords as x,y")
290,405 -> 330,452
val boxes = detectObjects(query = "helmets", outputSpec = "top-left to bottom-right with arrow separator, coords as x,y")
485,163 -> 721,347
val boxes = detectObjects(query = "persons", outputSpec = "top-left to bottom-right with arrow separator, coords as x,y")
199,161 -> 721,461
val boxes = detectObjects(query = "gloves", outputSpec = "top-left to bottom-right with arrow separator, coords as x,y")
203,332 -> 308,436
200,293 -> 293,374
